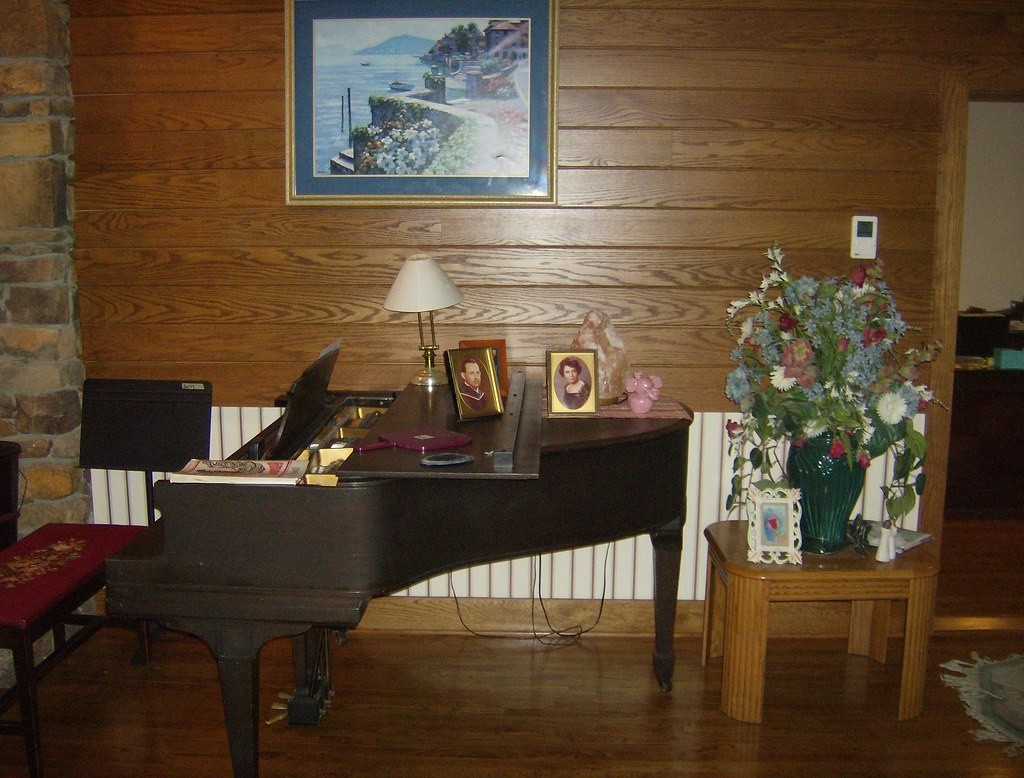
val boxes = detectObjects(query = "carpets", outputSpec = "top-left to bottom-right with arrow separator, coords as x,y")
937,654 -> 1024,761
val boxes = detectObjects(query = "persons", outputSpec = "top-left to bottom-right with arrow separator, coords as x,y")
458,358 -> 489,410
559,357 -> 591,409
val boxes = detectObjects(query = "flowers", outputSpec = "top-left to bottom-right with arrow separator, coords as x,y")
724,239 -> 949,524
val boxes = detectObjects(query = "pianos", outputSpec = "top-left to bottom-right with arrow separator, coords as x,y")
105,351 -> 693,778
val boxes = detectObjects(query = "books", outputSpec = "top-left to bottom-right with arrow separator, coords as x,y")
171,459 -> 309,486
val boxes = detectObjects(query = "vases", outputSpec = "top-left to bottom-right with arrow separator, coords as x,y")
786,431 -> 867,552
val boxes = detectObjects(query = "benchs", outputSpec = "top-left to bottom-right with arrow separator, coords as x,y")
0,524 -> 147,778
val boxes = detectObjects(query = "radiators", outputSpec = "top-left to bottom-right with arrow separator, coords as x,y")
90,406 -> 925,603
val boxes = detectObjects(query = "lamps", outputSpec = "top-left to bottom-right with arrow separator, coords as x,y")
382,253 -> 463,386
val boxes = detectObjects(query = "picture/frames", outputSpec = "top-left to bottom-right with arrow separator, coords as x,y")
458,338 -> 509,399
284,0 -> 559,208
444,347 -> 505,421
745,484 -> 804,565
546,349 -> 600,415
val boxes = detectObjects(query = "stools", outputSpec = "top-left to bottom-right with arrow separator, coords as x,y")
702,520 -> 940,725
